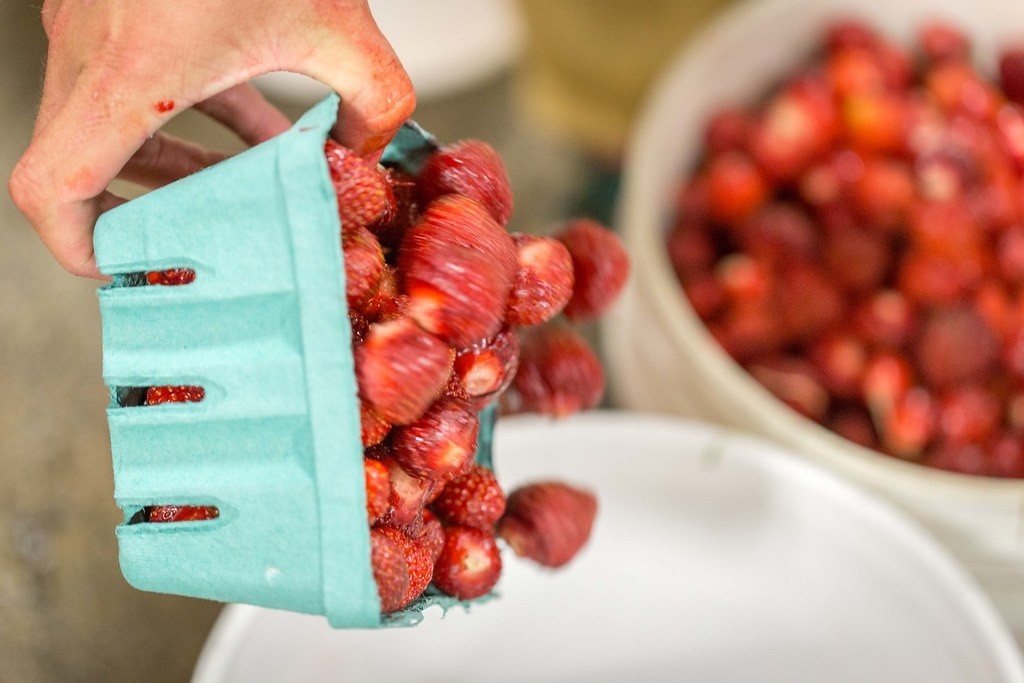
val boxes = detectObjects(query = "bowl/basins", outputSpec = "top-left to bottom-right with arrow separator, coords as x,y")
600,0 -> 1023,559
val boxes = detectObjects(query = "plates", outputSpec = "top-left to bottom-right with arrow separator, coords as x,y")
185,407 -> 1023,683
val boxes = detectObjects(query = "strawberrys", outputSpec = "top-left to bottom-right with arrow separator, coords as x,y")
663,20 -> 1024,483
127,138 -> 632,610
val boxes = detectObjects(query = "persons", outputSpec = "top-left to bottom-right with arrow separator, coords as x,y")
10,0 -> 418,282
